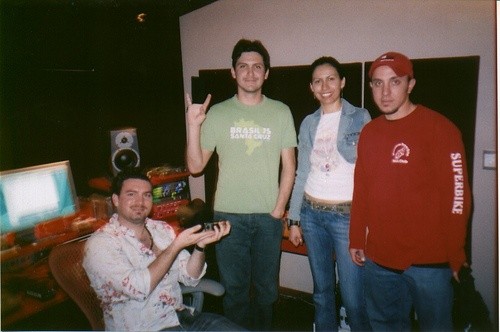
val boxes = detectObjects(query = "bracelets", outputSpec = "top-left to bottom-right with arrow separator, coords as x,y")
194,243 -> 209,252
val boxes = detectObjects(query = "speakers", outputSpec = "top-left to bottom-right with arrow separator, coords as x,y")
106,127 -> 140,178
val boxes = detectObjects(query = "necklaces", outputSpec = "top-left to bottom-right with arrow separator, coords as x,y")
318,100 -> 342,173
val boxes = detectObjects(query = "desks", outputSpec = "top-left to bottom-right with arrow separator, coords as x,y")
279,236 -> 339,301
0,199 -> 185,332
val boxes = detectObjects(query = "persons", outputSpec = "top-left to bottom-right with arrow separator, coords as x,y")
349,52 -> 471,332
82,170 -> 250,332
286,56 -> 373,331
186,39 -> 298,331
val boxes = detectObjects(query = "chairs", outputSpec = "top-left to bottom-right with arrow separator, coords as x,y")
48,244 -> 225,332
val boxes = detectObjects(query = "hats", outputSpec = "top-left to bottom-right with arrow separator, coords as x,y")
370,51 -> 415,80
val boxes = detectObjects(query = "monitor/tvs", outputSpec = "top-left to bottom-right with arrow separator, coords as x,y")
0,160 -> 81,247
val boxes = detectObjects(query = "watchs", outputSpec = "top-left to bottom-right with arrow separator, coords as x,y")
287,218 -> 301,227
